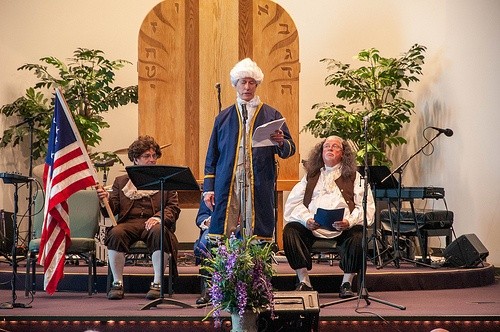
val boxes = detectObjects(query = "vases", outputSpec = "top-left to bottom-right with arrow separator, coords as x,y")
230,311 -> 259,332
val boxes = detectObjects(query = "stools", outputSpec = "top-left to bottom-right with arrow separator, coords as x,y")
381,208 -> 453,262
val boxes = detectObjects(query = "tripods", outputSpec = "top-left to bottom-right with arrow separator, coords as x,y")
126,166 -> 200,311
319,129 -> 441,310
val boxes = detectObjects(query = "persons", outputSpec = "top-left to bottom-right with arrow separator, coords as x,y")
95,135 -> 181,299
283,136 -> 375,298
193,58 -> 295,307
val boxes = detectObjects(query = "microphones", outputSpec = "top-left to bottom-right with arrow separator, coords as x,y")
432,127 -> 453,137
364,116 -> 368,130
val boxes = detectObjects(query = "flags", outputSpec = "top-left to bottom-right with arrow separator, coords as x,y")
36,88 -> 96,295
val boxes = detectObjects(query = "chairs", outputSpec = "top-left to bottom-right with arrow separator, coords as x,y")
107,241 -> 174,299
25,190 -> 99,297
311,239 -> 366,295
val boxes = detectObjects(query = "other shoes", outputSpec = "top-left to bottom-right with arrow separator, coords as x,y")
196,294 -> 212,308
108,280 -> 125,299
339,282 -> 354,298
294,282 -> 313,291
145,281 -> 161,299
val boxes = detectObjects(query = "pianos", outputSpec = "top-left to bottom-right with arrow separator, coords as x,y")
357,165 -> 445,267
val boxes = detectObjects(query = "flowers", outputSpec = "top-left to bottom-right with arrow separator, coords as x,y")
193,224 -> 279,320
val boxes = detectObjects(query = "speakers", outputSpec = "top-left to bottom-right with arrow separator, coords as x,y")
255,291 -> 320,332
442,233 -> 489,267
